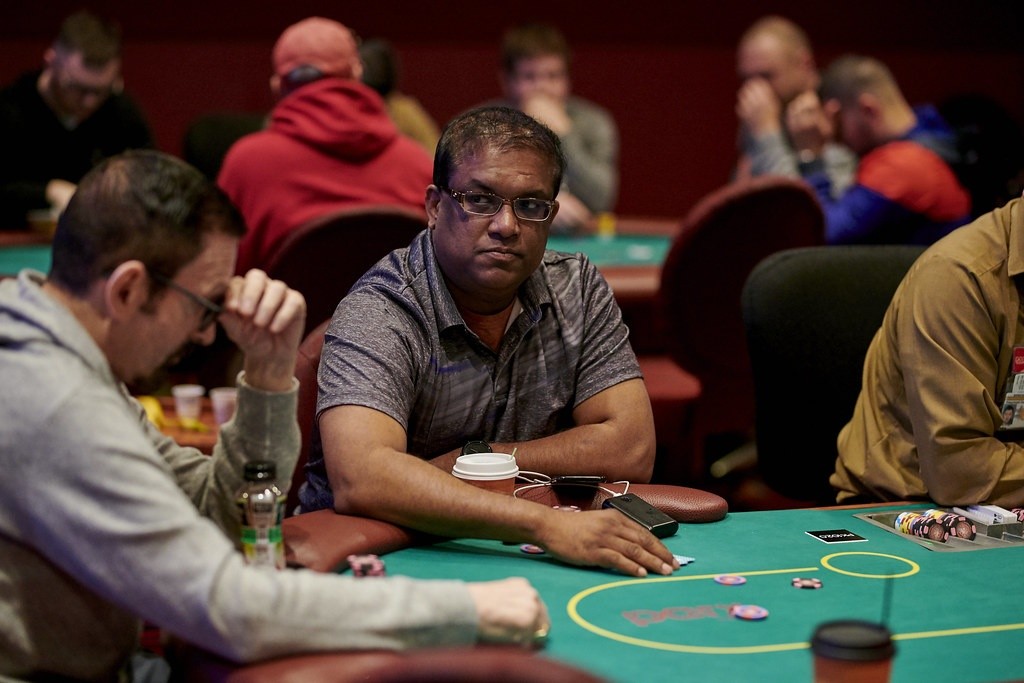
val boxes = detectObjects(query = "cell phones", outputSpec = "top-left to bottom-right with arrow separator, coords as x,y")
552,474 -> 607,484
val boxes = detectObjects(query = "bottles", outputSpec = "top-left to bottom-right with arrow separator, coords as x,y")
234,460 -> 285,570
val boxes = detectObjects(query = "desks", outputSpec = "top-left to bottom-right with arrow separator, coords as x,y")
156,498 -> 1024,683
546,216 -> 679,312
0,219 -> 58,282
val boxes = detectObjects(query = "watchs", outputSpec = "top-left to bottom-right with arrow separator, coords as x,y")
462,441 -> 491,457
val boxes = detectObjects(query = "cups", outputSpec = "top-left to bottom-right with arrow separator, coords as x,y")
811,620 -> 895,683
453,452 -> 519,497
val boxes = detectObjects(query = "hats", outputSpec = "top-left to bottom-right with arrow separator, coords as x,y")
272,17 -> 362,80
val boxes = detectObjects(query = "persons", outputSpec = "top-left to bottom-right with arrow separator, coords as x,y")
499,23 -> 619,234
829,192 -> 1024,507
728,15 -> 979,249
357,40 -> 444,156
0,13 -> 155,235
0,149 -> 551,683
213,14 -> 434,280
1002,405 -> 1014,424
314,102 -> 679,582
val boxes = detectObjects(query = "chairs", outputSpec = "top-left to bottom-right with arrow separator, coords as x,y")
259,203 -> 428,343
656,174 -> 832,483
742,245 -> 924,507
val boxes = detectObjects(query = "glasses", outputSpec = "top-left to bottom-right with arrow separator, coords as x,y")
57,55 -> 117,103
438,182 -> 557,223
101,265 -> 228,333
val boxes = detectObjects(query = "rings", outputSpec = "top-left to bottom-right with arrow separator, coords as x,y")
535,630 -> 548,642
